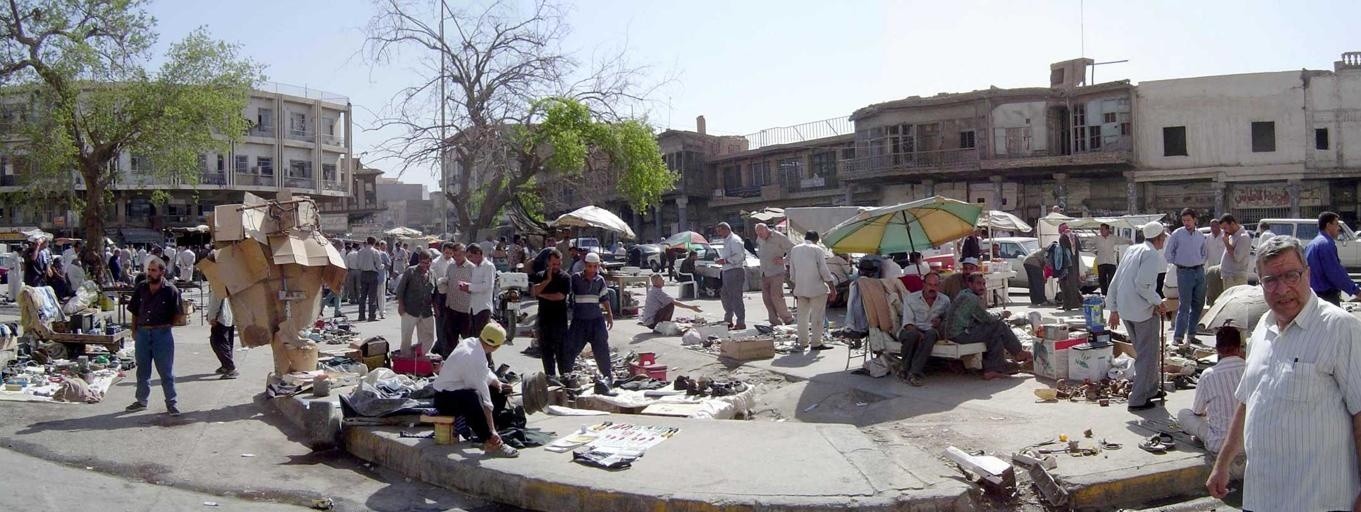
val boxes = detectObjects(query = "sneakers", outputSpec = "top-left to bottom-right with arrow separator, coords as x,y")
167,404 -> 181,415
216,367 -> 240,379
126,402 -> 148,412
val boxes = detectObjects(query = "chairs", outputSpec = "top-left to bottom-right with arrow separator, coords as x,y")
673,266 -> 699,299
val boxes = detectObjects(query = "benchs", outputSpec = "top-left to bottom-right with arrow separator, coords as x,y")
886,340 -> 987,375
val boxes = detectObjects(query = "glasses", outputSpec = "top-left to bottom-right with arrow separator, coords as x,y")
1257,270 -> 1301,293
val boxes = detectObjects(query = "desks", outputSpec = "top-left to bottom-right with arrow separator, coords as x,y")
102,281 -> 203,326
54,329 -> 128,355
604,274 -> 649,316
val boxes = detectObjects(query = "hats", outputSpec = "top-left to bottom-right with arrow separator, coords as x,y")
1143,221 -> 1164,239
584,253 -> 600,263
963,257 -> 978,267
479,322 -> 506,346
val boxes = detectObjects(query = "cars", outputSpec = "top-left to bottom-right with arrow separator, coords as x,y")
675,245 -> 755,261
982,237 -> 1101,292
558,238 -> 669,272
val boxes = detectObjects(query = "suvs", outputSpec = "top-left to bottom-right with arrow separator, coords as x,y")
1253,219 -> 1361,270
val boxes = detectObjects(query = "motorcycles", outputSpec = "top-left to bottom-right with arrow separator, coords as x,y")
494,270 -> 527,341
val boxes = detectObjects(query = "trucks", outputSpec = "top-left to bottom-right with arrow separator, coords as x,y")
1080,223 -> 1172,266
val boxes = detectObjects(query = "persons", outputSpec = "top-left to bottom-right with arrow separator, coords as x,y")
1176,324 -> 1247,455
432,321 -> 515,447
1303,211 -> 1361,309
321,234 -> 614,379
842,230 -> 1032,386
614,222 -> 837,350
1254,223 -> 1276,283
1023,209 -> 1252,411
22,235 -> 239,418
1201,234 -> 1361,512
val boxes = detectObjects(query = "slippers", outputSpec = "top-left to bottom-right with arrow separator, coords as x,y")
485,443 -> 519,457
1139,430 -> 1176,452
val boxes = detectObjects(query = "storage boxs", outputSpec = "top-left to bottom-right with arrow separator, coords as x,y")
393,355 -> 441,375
173,302 -> 193,326
721,339 -> 774,361
344,340 -> 387,371
1033,335 -> 1086,381
500,272 -> 528,292
1068,346 -> 1112,382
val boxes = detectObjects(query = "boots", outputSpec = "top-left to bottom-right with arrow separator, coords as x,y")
594,376 -> 618,395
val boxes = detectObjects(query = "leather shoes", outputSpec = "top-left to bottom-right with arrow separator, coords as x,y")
1129,391 -> 1167,410
812,343 -> 833,350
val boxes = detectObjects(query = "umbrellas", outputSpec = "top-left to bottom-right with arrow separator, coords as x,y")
818,191 -> 985,281
